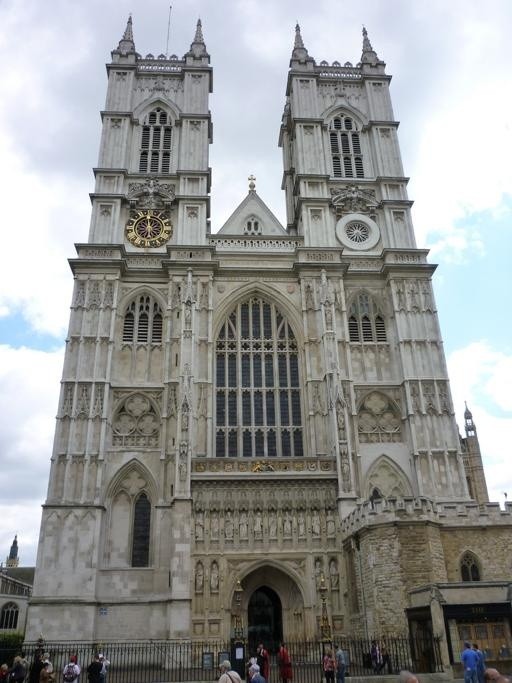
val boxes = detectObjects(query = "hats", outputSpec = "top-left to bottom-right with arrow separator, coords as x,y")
464,640 -> 471,647
98,653 -> 104,660
249,664 -> 260,674
70,655 -> 76,662
218,659 -> 232,669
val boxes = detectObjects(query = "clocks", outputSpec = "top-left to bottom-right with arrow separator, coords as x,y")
126,209 -> 173,248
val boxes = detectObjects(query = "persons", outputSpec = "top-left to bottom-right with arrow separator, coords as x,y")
256,647 -> 267,677
210,563 -> 219,590
472,643 -> 485,682
334,644 -> 346,683
97,653 -> 111,682
86,653 -> 103,682
247,664 -> 266,682
277,641 -> 292,682
399,669 -> 418,683
323,648 -> 336,682
62,655 -> 82,682
371,640 -> 382,674
0,651 -> 57,682
217,659 -> 242,683
482,668 -> 500,682
195,564 -> 203,590
314,561 -> 323,583
195,508 -> 335,538
329,560 -> 339,586
373,634 -> 394,674
260,641 -> 270,682
461,641 -> 480,682
